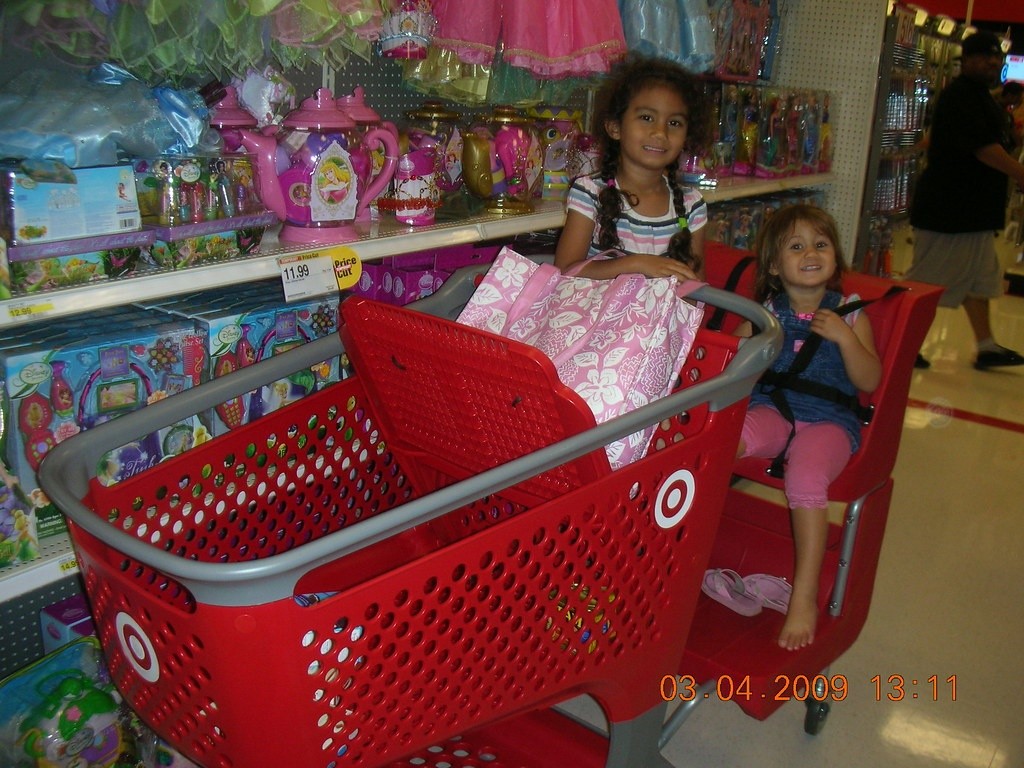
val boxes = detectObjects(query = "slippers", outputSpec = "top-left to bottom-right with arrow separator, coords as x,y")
742,574 -> 793,615
700,568 -> 762,617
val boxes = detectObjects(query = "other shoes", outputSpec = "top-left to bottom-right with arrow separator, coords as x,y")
977,347 -> 1024,366
914,352 -> 930,368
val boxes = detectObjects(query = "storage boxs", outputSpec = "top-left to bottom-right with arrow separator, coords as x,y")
346,243 -> 513,302
0,280 -> 343,536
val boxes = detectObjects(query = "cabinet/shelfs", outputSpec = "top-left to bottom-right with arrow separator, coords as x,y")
852,8 -> 975,277
0,168 -> 834,624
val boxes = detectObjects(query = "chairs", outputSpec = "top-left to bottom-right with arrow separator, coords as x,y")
702,240 -> 946,502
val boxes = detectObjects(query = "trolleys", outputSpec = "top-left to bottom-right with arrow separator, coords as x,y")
32,250 -> 786,768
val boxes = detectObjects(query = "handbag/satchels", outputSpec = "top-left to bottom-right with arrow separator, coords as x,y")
455,250 -> 710,501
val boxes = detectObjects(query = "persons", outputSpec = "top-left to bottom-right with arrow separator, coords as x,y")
900,28 -> 1024,368
707,204 -> 882,651
554,53 -> 708,282
692,87 -> 833,167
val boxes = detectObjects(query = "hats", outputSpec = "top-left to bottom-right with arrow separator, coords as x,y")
952,33 -> 1003,61
